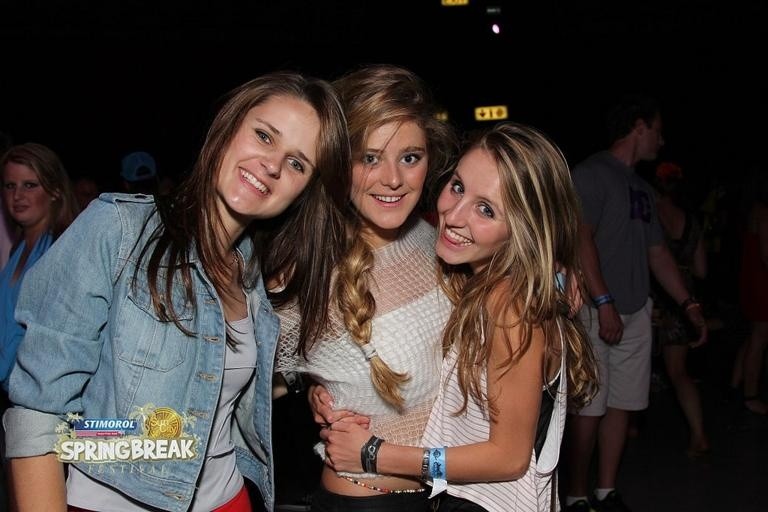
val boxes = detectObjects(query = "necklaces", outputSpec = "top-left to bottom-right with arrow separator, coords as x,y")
227,249 -> 238,267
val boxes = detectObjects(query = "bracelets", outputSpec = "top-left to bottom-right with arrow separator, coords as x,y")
421,447 -> 446,483
679,297 -> 699,311
593,293 -> 614,306
361,435 -> 385,474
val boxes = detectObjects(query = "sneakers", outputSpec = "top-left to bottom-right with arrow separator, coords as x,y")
564,499 -> 595,512
588,490 -> 631,512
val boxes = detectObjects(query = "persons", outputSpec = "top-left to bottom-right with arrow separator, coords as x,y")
307,121 -> 602,512
262,67 -> 468,511
627,161 -> 709,458
0,143 -> 80,396
729,191 -> 768,418
70,150 -> 189,208
562,94 -> 709,511
2,74 -> 353,512
